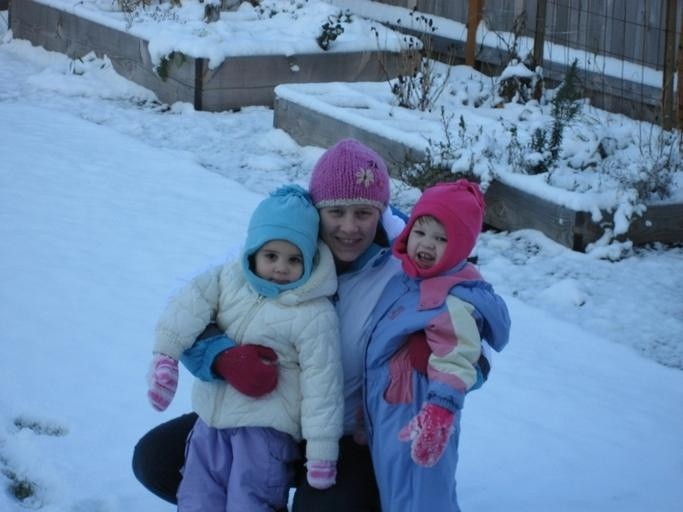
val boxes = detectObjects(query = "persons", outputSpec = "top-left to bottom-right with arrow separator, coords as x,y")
146,184 -> 345,511
359,178 -> 511,512
133,137 -> 494,511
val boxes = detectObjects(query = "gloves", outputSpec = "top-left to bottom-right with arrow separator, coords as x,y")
306,459 -> 337,489
401,402 -> 454,467
147,353 -> 180,411
409,331 -> 429,375
214,344 -> 278,397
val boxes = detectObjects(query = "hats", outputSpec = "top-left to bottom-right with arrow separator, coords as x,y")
311,138 -> 390,214
393,180 -> 484,278
241,183 -> 320,298
